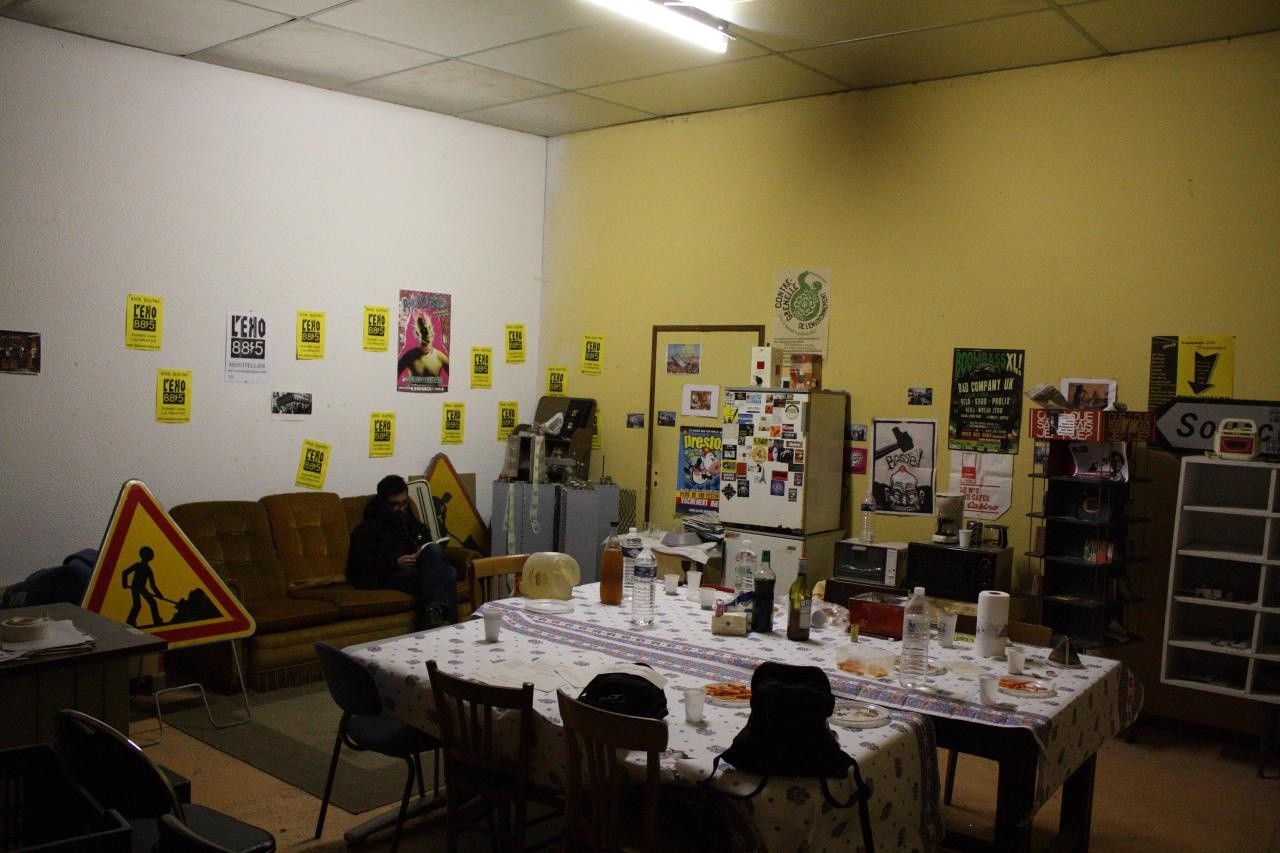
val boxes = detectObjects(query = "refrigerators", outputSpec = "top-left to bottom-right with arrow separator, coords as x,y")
719,386 -> 848,601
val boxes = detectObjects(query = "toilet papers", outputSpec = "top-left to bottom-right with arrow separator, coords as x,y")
974,590 -> 1013,658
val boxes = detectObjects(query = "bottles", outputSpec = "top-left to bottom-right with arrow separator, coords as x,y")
735,539 -> 813,643
598,521 -> 657,627
967,513 -> 983,546
898,587 -> 932,688
565,474 -> 593,490
859,490 -> 876,544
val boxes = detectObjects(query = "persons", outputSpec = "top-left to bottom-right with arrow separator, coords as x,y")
349,473 -> 459,630
398,312 -> 449,390
1088,451 -> 1125,482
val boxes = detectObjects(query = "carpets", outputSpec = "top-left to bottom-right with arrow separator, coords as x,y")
136,683 -> 445,814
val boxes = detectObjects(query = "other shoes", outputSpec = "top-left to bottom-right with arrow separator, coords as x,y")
425,607 -> 447,626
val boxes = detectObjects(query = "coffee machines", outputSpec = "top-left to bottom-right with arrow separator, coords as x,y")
932,492 -> 966,543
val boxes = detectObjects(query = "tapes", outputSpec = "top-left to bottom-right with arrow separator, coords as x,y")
0,616 -> 47,642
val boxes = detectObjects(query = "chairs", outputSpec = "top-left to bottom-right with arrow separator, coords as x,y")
844,598 -> 906,641
55,708 -> 276,853
426,660 -> 566,853
944,615 -> 1052,804
556,690 -> 700,853
313,641 -> 443,853
469,553 -> 529,613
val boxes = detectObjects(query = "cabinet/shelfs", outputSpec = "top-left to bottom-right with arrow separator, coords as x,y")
1161,457 -> 1280,708
1023,437 -> 1153,653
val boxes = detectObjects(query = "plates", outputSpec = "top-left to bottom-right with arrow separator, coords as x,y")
828,701 -> 890,728
998,675 -> 1056,697
894,658 -> 945,674
699,683 -> 751,704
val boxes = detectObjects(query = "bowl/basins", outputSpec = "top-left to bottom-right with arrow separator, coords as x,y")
835,642 -> 896,677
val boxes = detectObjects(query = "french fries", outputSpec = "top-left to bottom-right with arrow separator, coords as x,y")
998,679 -> 1034,691
703,683 -> 752,699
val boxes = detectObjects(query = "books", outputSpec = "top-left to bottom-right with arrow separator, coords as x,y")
414,536 -> 450,556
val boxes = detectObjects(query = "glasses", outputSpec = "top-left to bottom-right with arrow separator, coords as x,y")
386,502 -> 408,510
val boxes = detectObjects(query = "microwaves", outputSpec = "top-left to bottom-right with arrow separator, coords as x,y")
833,538 -> 909,588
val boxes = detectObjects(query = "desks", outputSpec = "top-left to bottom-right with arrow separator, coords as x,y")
0,600 -> 167,756
336,619 -> 943,853
484,575 -> 1150,853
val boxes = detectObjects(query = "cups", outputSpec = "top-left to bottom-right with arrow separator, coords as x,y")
959,529 -> 972,547
665,574 -> 680,595
1006,646 -> 1026,675
699,587 -> 716,610
642,522 -> 662,538
483,608 -> 501,641
684,688 -> 706,722
979,674 -> 999,705
687,571 -> 702,591
936,612 -> 957,648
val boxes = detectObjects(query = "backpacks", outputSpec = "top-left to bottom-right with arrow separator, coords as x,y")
710,663 -> 869,810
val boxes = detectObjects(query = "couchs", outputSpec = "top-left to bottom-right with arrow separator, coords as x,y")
168,492 -> 485,692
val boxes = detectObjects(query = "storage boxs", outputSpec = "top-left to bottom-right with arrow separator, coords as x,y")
790,353 -> 823,390
751,347 -> 783,388
0,744 -> 134,853
